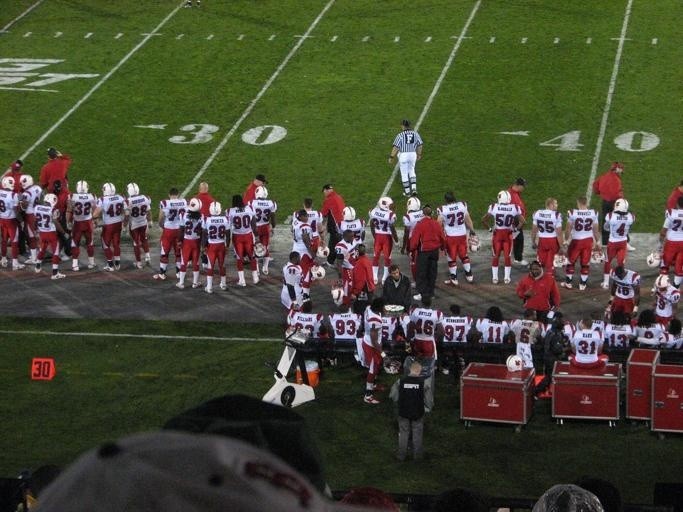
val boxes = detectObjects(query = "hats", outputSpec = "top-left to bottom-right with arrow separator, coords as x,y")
47,147 -> 57,155
256,174 -> 268,184
400,120 -> 410,126
322,184 -> 333,192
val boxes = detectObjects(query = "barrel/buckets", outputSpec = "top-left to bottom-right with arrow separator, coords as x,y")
297,360 -> 321,387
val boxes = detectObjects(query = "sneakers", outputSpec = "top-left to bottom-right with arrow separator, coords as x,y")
444,257 -> 529,289
403,190 -> 418,197
363,394 -> 382,405
627,244 -> 636,251
153,266 -> 269,294
561,282 -> 610,290
413,293 -> 422,301
1,251 -> 151,281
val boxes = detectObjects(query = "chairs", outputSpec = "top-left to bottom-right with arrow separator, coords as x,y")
299,338 -> 683,385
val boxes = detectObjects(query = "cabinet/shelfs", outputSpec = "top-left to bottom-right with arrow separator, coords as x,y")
651,363 -> 682,439
458,362 -> 535,435
623,348 -> 660,427
550,358 -> 622,430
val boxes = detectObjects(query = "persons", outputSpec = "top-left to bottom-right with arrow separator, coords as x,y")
388,121 -> 423,197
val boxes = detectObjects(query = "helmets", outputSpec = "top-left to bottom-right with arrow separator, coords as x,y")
253,242 -> 269,258
76,180 -> 140,196
497,190 -> 511,204
553,254 -> 568,268
383,359 -> 402,375
255,186 -> 268,199
469,237 -> 480,252
189,197 -> 202,212
379,197 -> 394,211
2,174 -> 59,209
209,201 -> 221,216
407,197 -> 421,212
647,253 -> 669,291
590,251 -> 606,265
506,355 -> 523,372
311,245 -> 331,280
342,206 -> 356,221
614,198 -> 629,213
